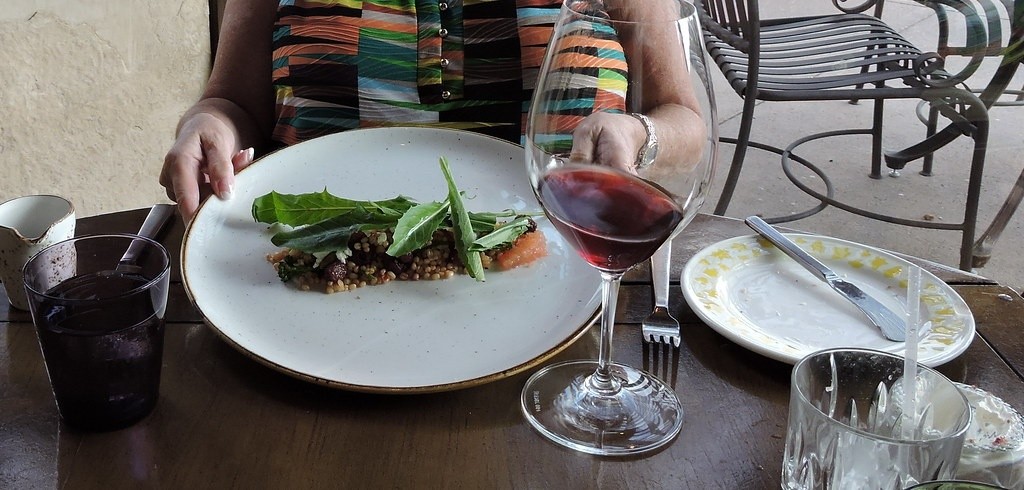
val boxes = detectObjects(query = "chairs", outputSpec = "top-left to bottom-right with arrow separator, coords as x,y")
691,0 -> 1024,273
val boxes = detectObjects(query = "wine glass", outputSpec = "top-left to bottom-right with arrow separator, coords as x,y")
522,1 -> 720,456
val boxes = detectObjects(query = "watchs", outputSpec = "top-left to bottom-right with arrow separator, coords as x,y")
625,112 -> 659,174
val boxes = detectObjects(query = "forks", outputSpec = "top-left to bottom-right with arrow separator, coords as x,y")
642,231 -> 680,348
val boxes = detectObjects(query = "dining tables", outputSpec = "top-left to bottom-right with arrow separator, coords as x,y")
0,202 -> 1024,490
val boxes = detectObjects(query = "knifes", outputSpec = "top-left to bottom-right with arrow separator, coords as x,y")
116,203 -> 175,273
744,215 -> 907,341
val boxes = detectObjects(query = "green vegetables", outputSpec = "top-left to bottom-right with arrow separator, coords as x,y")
251,158 -> 547,283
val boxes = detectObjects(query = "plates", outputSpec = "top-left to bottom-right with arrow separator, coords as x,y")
681,231 -> 976,373
179,126 -> 602,394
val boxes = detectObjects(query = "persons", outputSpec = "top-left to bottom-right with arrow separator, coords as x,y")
159,0 -> 707,230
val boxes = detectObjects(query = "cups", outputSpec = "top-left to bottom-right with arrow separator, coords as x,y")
0,194 -> 78,311
780,347 -> 973,490
21,233 -> 170,433
